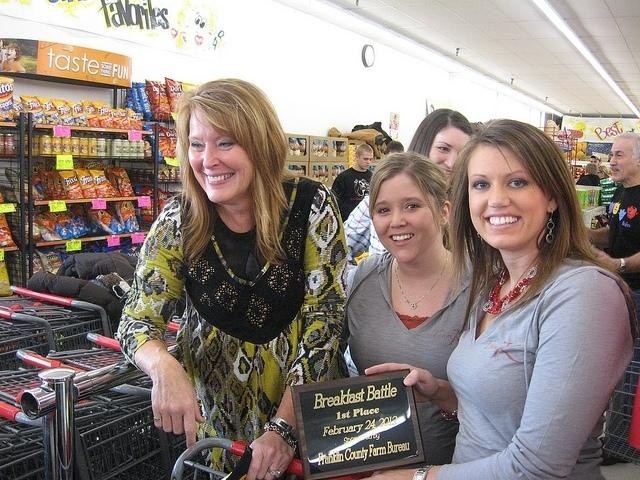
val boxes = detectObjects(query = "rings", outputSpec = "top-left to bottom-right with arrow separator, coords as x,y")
269,470 -> 281,477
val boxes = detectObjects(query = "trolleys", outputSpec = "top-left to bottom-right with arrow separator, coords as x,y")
1,269 -> 208,479
602,289 -> 640,467
169,437 -> 364,479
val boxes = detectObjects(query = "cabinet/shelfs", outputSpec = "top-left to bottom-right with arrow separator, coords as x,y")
1,102 -> 180,292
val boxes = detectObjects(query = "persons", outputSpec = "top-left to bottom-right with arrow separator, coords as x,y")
342,109 -> 474,258
590,156 -> 596,163
386,141 -> 404,154
331,144 -> 374,224
360,117 -> 636,480
342,153 -> 473,466
116,78 -> 349,480
584,131 -> 640,467
576,163 -> 600,186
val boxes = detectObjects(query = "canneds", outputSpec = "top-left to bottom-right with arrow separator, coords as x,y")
0,134 -> 144,160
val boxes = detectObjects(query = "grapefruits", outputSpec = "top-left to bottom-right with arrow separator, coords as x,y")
564,143 -> 587,161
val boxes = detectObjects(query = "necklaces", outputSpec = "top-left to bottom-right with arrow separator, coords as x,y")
392,255 -> 450,310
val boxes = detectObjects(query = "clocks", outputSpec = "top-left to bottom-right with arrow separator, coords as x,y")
362,43 -> 376,68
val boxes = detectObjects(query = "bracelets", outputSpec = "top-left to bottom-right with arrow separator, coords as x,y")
412,464 -> 433,480
619,258 -> 626,274
270,417 -> 298,437
264,422 -> 297,453
